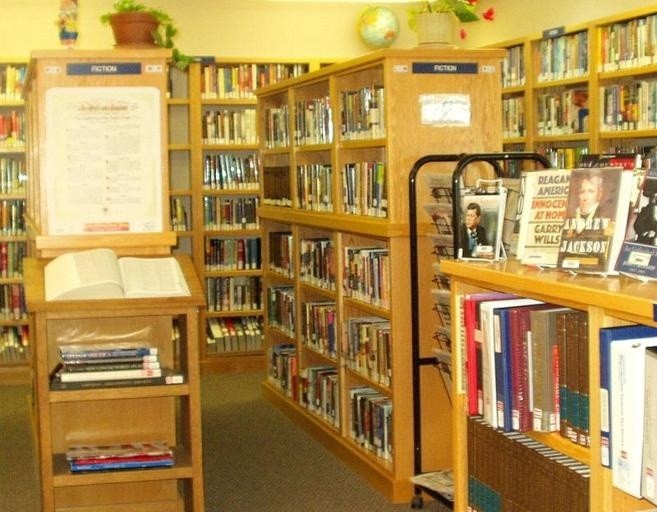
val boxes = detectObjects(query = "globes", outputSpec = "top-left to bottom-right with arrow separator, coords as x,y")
357,6 -> 399,50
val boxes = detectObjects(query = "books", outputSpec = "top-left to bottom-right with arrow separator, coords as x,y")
409,288 -> 656,511
424,13 -> 656,280
48,342 -> 185,391
43,248 -> 191,301
65,441 -> 175,474
265,84 -> 393,464
0,63 -> 29,363
166,62 -> 310,358
